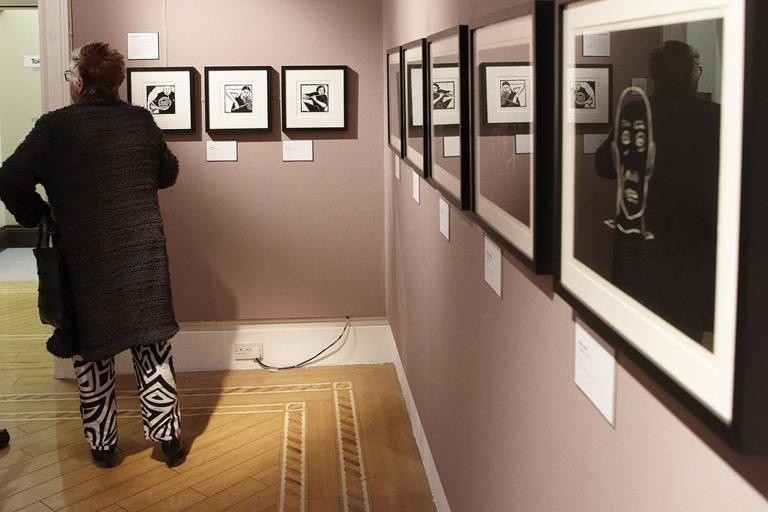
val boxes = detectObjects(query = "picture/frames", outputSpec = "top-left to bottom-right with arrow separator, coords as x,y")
553,1 -> 768,455
204,66 -> 274,134
385,46 -> 405,158
401,38 -> 428,179
468,1 -> 555,276
281,66 -> 348,132
427,25 -> 470,212
126,66 -> 195,135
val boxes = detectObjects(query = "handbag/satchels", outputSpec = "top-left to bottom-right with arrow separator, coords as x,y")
32,248 -> 74,330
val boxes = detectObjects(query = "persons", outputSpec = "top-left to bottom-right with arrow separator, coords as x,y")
231,86 -> 252,112
302,86 -> 327,112
594,39 -> 720,344
500,81 -> 525,107
432,83 -> 453,109
571,81 -> 596,109
149,86 -> 175,114
0,41 -> 188,468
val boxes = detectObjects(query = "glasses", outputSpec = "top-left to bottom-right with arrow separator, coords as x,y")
64,70 -> 74,81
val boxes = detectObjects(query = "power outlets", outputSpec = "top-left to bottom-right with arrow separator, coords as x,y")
234,343 -> 263,359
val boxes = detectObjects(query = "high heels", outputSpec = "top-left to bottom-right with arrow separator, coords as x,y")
162,437 -> 186,466
91,442 -> 117,468
